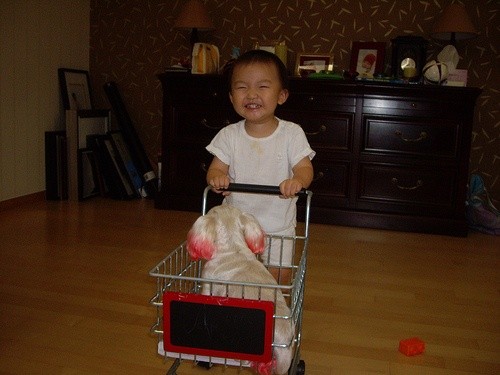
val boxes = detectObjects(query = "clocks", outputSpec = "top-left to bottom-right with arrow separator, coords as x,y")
391,35 -> 427,85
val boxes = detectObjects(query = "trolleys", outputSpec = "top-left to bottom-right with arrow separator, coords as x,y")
149,181 -> 313,375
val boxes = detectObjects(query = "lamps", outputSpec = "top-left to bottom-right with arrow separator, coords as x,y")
429,6 -> 475,45
174,0 -> 215,55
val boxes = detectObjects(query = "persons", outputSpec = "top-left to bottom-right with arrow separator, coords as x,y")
360,53 -> 376,73
206,48 -> 316,306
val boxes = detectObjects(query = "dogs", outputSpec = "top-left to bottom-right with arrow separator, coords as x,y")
185,205 -> 296,375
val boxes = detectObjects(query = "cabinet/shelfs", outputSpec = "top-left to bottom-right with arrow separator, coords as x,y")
157,74 -> 483,238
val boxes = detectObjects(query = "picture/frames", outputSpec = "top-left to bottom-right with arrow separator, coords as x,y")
295,52 -> 334,77
350,42 -> 386,77
45,68 -> 147,200
251,39 -> 285,56
442,64 -> 472,88
